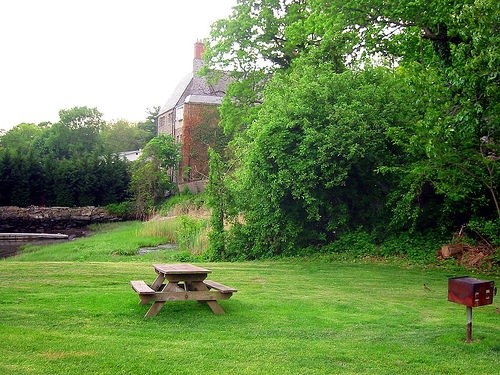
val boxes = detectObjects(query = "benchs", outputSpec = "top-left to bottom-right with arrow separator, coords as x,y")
131,280 -> 156,295
203,280 -> 238,294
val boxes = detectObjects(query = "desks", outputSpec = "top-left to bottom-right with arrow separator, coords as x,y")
138,264 -> 226,321
